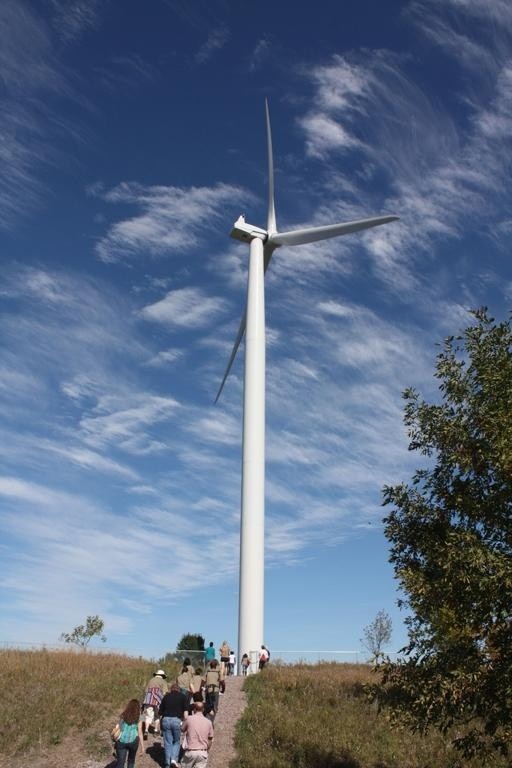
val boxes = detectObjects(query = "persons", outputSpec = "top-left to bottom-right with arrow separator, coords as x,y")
241,653 -> 252,676
259,645 -> 268,670
180,701 -> 214,768
141,669 -> 168,740
220,640 -> 229,675
176,641 -> 225,716
159,684 -> 188,768
228,651 -> 236,676
111,699 -> 145,768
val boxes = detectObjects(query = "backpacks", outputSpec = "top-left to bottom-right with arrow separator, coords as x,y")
260,654 -> 267,663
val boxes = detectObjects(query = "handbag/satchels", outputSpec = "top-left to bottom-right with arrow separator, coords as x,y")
110,728 -> 122,742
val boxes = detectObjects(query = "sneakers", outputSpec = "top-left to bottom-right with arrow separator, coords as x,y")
143,731 -> 149,741
153,729 -> 160,735
171,759 -> 179,768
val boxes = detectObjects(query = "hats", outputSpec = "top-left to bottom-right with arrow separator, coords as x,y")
151,669 -> 167,678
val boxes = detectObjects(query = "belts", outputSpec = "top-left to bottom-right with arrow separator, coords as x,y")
187,748 -> 208,752
